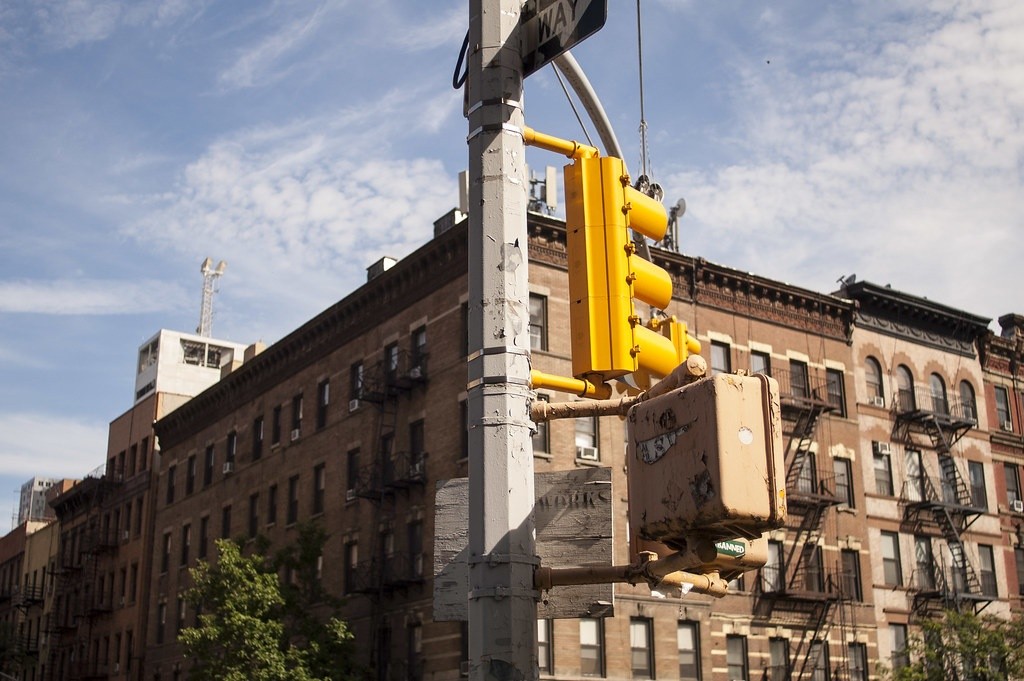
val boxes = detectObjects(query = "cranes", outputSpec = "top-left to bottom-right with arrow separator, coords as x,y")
196,257 -> 227,337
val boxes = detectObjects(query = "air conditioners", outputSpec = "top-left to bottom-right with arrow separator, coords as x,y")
347,489 -> 356,501
575,446 -> 598,460
869,396 -> 884,408
114,663 -> 119,672
873,441 -> 889,455
122,530 -> 129,540
223,462 -> 233,474
119,597 -> 124,605
349,399 -> 360,412
291,429 -> 301,442
410,463 -> 423,477
410,367 -> 422,379
1009,500 -> 1024,512
1000,420 -> 1012,432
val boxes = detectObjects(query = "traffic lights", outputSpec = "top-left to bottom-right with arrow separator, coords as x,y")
567,155 -> 678,379
663,316 -> 702,363
